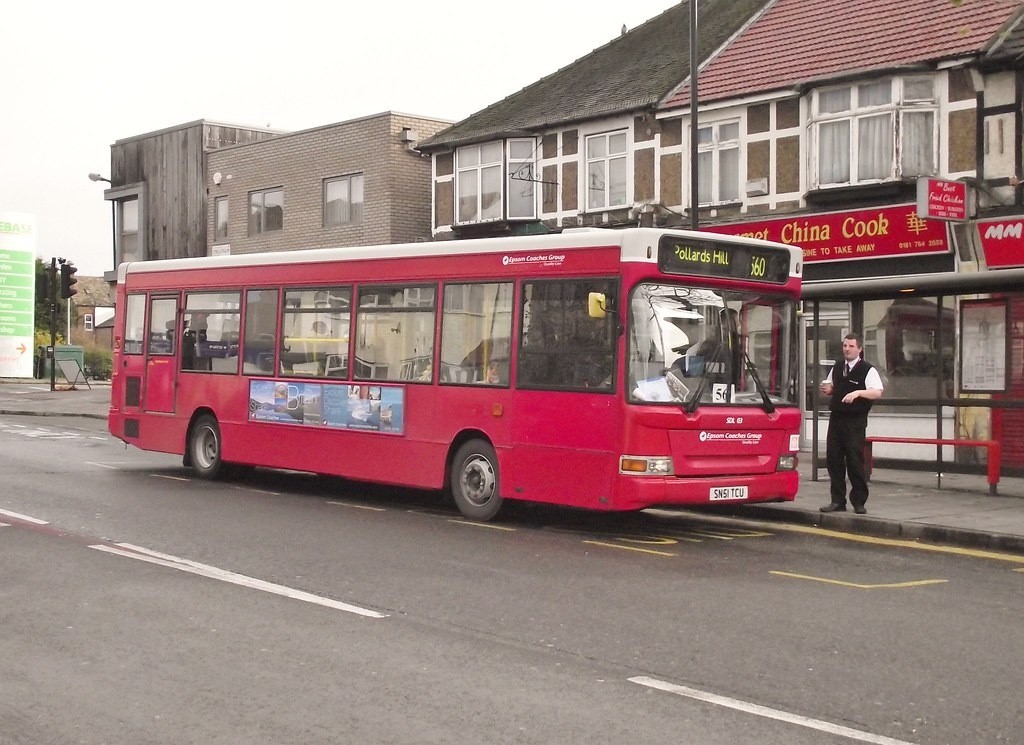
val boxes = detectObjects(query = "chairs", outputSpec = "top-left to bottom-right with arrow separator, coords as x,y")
148,328 -> 341,376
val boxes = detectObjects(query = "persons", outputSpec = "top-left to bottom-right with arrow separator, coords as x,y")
695,308 -> 741,391
819,332 -> 884,514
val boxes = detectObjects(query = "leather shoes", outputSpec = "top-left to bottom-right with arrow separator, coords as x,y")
854,505 -> 866,514
819,501 -> 846,512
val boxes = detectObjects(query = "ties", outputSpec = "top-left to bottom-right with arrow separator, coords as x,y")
845,363 -> 850,376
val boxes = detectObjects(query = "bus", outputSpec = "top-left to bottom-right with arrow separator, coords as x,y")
107,226 -> 803,523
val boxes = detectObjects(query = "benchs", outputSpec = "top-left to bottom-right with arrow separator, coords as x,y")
866,436 -> 1002,494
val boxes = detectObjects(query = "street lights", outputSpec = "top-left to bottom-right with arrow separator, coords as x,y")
87,173 -> 116,270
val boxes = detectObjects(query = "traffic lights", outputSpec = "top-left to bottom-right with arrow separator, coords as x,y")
60,264 -> 78,299
